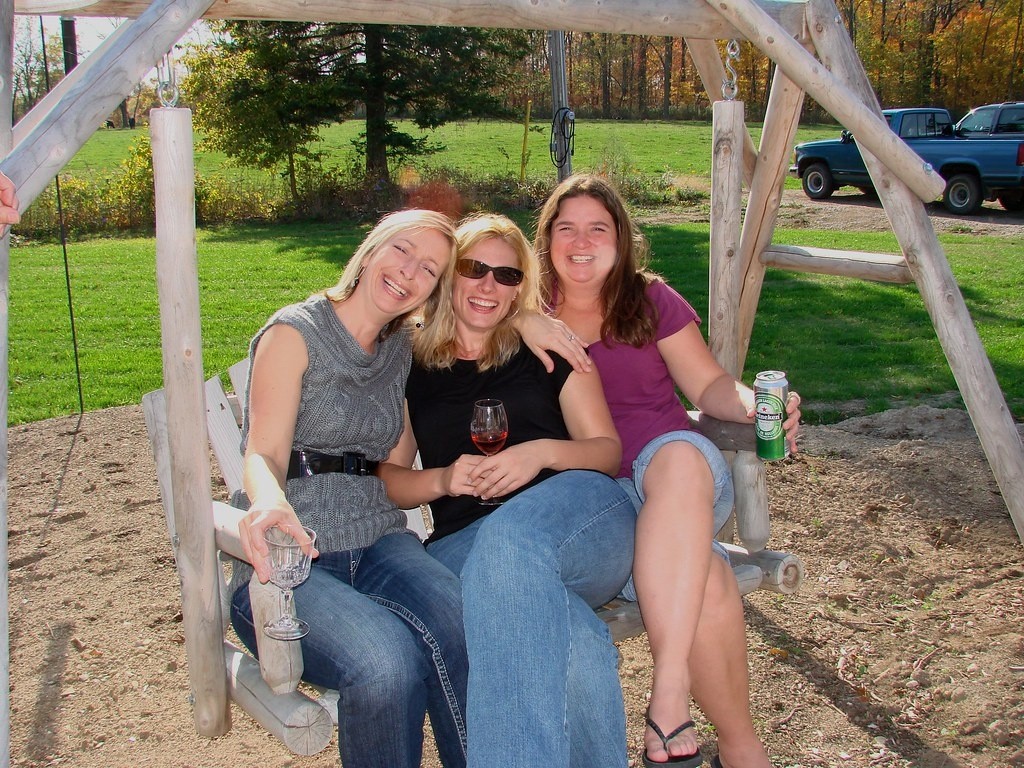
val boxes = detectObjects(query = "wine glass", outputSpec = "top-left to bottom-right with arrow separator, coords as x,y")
472,400 -> 507,506
263,525 -> 315,641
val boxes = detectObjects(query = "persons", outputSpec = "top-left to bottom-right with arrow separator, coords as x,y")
226,209 -> 637,768
0,170 -> 21,238
531,175 -> 801,768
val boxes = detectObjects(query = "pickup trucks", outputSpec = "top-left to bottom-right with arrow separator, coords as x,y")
785,106 -> 1024,216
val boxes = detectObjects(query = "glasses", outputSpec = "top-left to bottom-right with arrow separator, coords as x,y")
455,259 -> 524,286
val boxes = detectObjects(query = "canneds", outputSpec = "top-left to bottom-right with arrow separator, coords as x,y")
754,370 -> 792,461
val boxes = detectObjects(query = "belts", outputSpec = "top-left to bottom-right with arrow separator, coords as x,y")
286,451 -> 379,480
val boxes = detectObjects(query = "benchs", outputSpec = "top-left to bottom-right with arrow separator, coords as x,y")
142,357 -> 804,756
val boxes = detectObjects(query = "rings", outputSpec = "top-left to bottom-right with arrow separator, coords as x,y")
568,333 -> 575,341
789,392 -> 800,406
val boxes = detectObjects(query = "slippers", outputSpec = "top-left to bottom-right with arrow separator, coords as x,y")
642,704 -> 701,768
710,755 -> 723,768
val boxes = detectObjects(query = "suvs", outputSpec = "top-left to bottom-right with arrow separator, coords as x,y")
918,102 -> 1024,135
